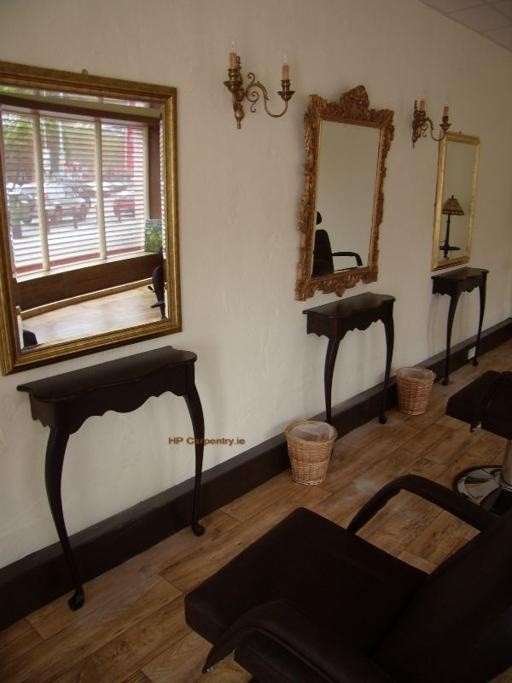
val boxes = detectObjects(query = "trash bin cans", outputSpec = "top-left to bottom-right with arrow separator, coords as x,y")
396,368 -> 436,414
285,420 -> 338,485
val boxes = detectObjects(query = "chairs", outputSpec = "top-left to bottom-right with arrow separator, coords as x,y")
313,212 -> 363,273
148,262 -> 166,317
183,476 -> 511,682
22,328 -> 39,347
446,370 -> 512,516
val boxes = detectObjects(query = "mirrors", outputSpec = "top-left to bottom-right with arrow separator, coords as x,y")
431,130 -> 481,271
0,58 -> 183,375
296,83 -> 396,301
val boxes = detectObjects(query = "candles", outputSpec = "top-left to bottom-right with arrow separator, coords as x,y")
281,49 -> 290,80
227,39 -> 239,68
442,105 -> 450,125
414,99 -> 427,112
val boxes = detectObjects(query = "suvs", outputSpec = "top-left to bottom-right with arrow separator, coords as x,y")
18,181 -> 89,225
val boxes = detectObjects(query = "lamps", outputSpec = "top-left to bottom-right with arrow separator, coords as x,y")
440,193 -> 465,257
